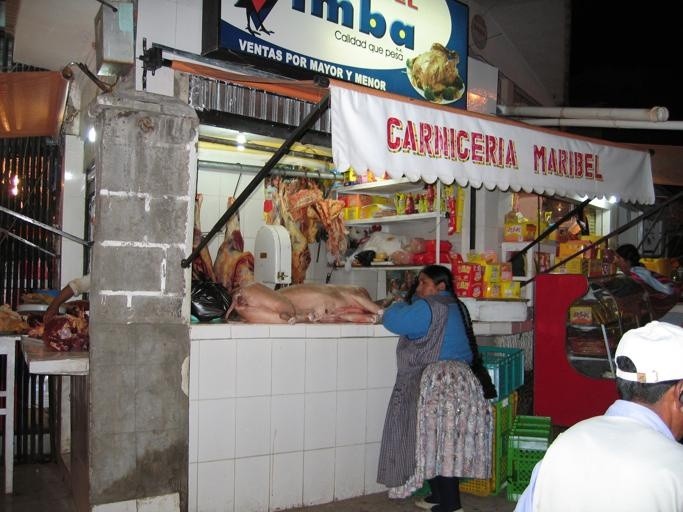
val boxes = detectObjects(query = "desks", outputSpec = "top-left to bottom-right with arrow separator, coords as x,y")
0,328 -> 27,501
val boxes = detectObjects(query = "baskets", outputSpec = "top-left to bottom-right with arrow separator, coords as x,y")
459,346 -> 551,503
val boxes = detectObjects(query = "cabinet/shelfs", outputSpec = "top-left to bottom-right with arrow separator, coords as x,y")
327,174 -> 462,277
502,238 -> 557,310
563,284 -> 654,381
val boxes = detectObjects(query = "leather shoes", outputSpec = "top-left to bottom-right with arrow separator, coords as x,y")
416,498 -> 463,512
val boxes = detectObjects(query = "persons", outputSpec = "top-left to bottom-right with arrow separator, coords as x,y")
42,274 -> 89,325
615,243 -> 682,318
512,319 -> 682,512
376,264 -> 496,512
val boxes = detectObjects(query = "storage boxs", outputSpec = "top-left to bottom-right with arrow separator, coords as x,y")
502,222 -> 674,276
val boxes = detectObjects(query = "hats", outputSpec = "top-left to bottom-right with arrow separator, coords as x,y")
614,319 -> 683,383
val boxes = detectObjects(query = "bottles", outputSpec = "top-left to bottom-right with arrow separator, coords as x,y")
347,168 -> 374,187
548,223 -> 559,245
396,190 -> 413,216
